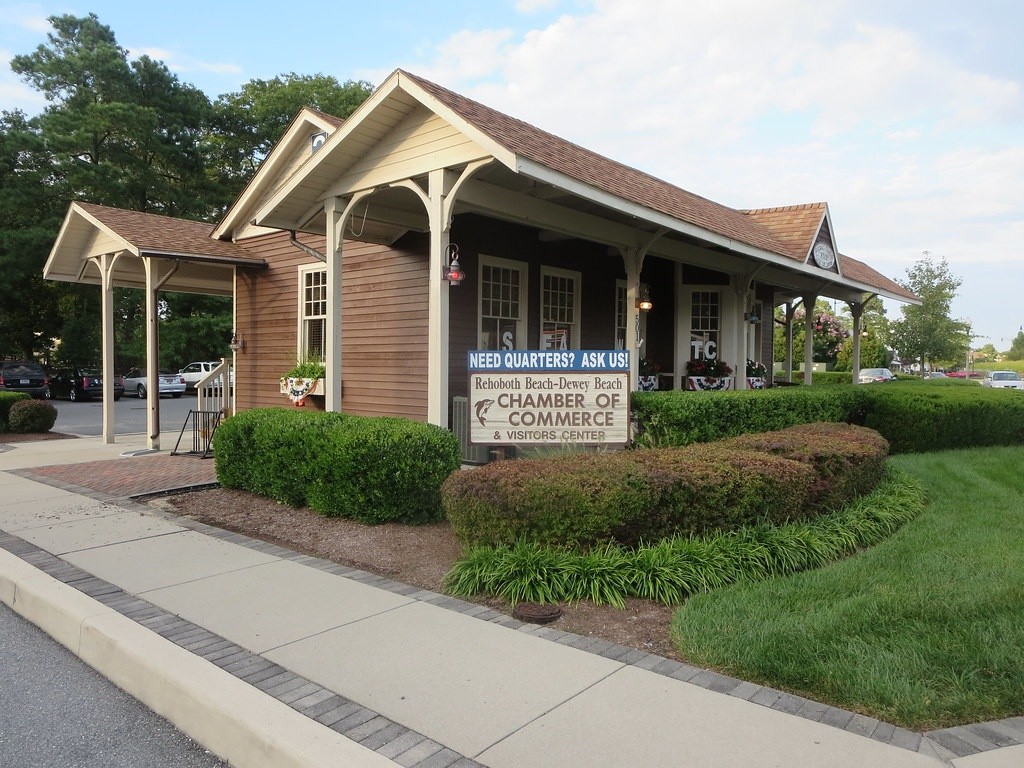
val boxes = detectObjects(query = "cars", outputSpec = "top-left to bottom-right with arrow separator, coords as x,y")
857,366 -> 898,385
983,371 -> 1024,389
922,369 -> 948,382
1,360 -> 50,400
177,360 -> 234,397
119,364 -> 189,397
49,365 -> 127,401
